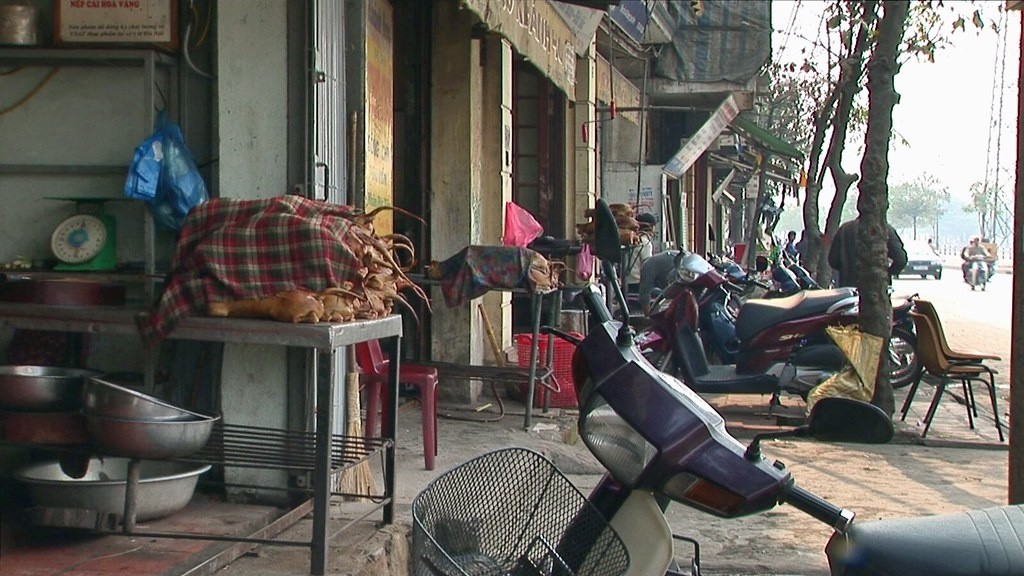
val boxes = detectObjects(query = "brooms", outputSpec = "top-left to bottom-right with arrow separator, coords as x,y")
477,304 -> 520,400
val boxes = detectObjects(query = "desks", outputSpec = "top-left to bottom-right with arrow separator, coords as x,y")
0,302 -> 404,576
399,274 -> 558,430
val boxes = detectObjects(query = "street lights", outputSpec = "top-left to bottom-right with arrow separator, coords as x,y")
998,1 -> 1023,508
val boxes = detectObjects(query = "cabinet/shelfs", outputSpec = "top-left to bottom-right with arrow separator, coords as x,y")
0,49 -> 189,304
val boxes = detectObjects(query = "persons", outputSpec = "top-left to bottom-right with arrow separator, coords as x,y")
827,201 -> 908,289
961,233 -> 993,279
927,238 -> 935,250
785,230 -> 805,264
625,213 -> 653,294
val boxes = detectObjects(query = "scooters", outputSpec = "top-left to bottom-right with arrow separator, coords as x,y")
404,199 -> 1023,576
613,221 -> 923,402
961,255 -> 990,291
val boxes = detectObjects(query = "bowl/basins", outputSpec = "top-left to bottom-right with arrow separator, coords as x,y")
84,412 -> 222,457
87,374 -> 213,420
14,458 -> 212,524
1,364 -> 88,459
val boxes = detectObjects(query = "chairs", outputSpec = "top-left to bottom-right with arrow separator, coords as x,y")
356,336 -> 441,470
900,300 -> 1004,442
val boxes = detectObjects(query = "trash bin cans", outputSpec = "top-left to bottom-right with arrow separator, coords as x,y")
513,332 -> 585,407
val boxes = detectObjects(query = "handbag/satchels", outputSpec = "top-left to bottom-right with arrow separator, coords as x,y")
576,243 -> 594,280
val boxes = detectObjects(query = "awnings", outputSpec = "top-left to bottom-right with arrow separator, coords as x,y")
729,110 -> 805,160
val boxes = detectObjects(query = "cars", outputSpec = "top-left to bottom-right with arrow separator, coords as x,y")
894,240 -> 946,280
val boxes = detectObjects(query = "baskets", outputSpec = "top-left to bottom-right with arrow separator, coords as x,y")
514,331 -> 585,407
412,447 -> 630,576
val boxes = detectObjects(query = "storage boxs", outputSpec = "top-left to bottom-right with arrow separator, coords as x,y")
53,0 -> 179,48
980,243 -> 996,257
558,309 -> 590,335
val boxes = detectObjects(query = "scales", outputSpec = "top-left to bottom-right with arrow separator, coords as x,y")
43,195 -> 138,270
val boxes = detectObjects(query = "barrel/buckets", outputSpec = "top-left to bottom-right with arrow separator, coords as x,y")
0,2 -> 40,47
